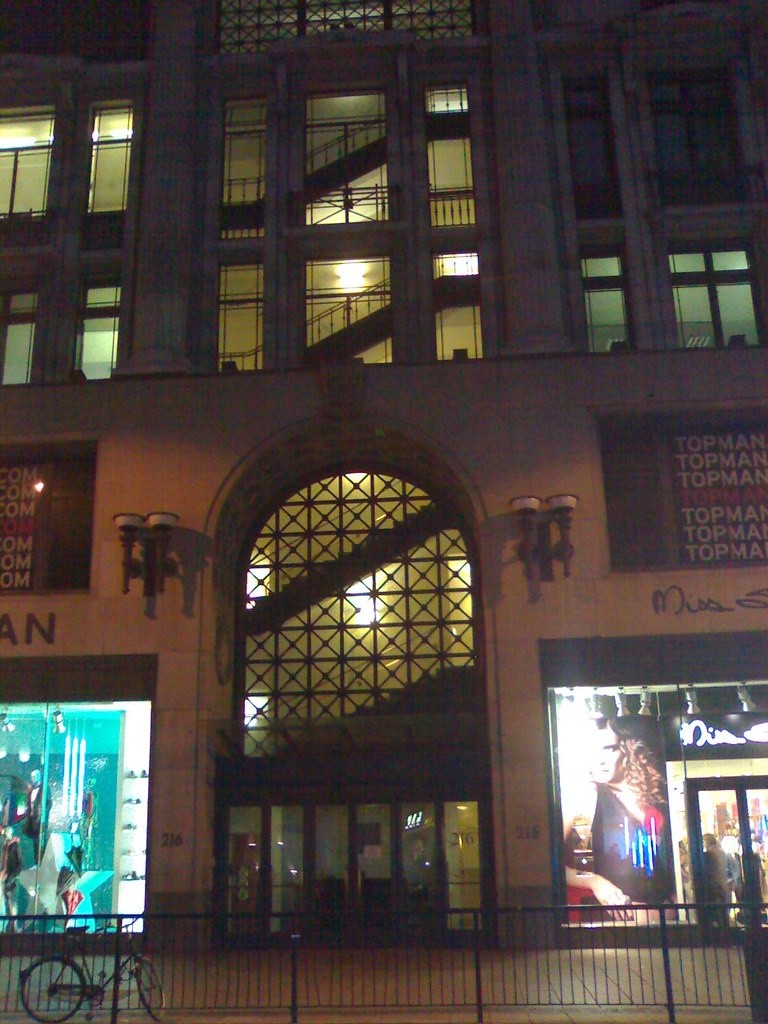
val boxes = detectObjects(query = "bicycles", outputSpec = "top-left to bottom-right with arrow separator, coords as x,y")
18,910 -> 166,1024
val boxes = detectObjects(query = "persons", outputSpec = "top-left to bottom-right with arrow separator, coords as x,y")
0,826 -> 23,933
564,713 -> 678,923
25,769 -> 53,864
678,827 -> 765,926
